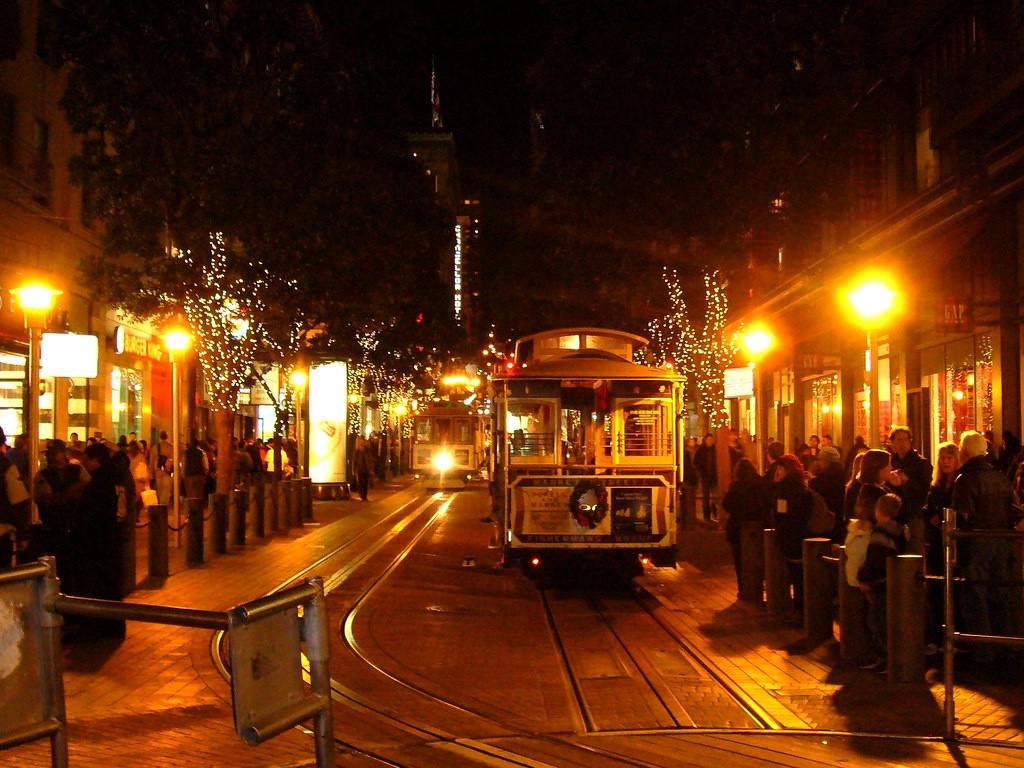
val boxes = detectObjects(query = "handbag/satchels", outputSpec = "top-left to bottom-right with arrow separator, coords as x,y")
803,485 -> 836,533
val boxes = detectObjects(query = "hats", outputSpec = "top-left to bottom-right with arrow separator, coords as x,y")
819,446 -> 840,462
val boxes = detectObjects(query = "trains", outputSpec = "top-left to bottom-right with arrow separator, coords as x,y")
488,327 -> 689,587
408,398 -> 494,491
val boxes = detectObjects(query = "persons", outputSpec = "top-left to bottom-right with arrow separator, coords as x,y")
0,423 -> 409,645
679,419 -> 1024,683
506,410 -> 646,473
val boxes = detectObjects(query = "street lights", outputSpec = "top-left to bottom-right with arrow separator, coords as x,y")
5,276 -> 68,523
742,323 -> 782,478
839,267 -> 900,450
157,325 -> 193,549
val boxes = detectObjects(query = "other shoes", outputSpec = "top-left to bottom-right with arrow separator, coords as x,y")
859,657 -> 888,674
